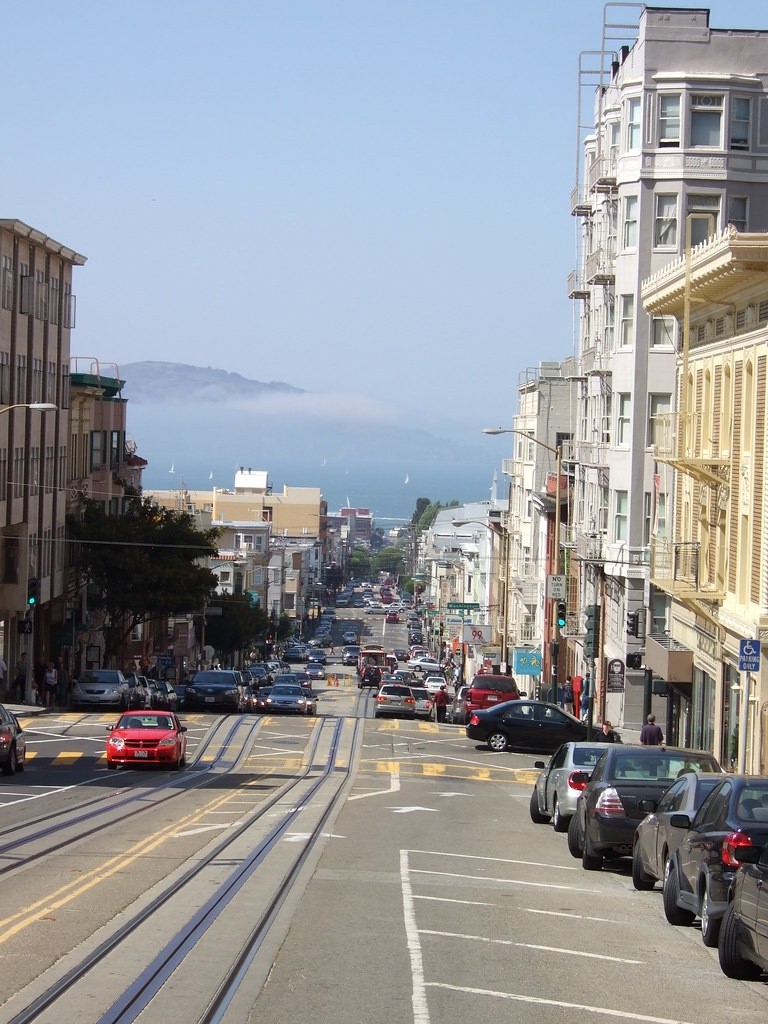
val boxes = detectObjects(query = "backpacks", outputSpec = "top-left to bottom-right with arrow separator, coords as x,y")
564,683 -> 574,702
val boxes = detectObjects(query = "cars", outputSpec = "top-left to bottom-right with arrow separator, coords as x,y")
631,771 -> 768,978
530,741 -> 621,832
568,744 -> 723,870
465,700 -> 624,754
124,577 -> 470,724
105,710 -> 188,770
465,673 -> 527,723
0,703 -> 27,774
71,669 -> 130,712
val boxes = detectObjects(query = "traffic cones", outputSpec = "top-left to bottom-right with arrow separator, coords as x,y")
334,678 -> 338,687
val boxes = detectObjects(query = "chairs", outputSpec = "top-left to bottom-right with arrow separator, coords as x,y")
618,766 -> 635,777
741,798 -> 761,821
677,769 -> 696,778
529,708 -> 533,719
129,719 -> 142,728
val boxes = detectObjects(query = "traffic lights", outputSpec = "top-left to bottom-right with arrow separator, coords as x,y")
557,600 -> 566,627
28,579 -> 38,605
627,654 -> 642,668
18,620 -> 33,634
584,605 -> 600,658
627,612 -> 638,637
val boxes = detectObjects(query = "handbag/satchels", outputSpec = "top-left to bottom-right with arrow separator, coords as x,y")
428,704 -> 434,718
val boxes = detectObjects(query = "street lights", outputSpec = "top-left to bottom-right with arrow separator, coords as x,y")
483,427 -> 561,703
452,520 -> 510,676
415,579 -> 437,652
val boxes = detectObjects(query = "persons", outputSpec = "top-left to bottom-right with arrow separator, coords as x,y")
16,653 -> 27,704
579,673 -> 590,723
34,656 -> 68,708
563,676 -> 574,715
0,660 -> 7,680
433,686 -> 449,724
593,721 -> 614,743
330,646 -> 335,655
640,714 -> 663,746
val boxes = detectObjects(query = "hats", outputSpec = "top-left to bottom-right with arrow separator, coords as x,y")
604,721 -> 611,726
